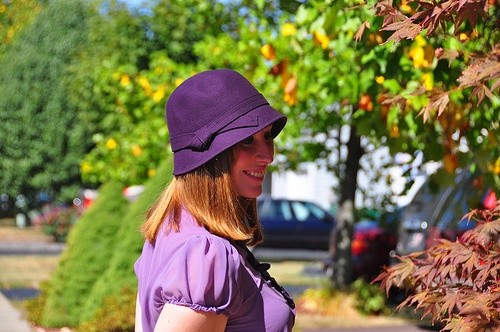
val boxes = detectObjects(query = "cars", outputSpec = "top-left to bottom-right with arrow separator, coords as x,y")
254,194 -> 335,251
350,210 -> 409,302
396,162 -> 500,259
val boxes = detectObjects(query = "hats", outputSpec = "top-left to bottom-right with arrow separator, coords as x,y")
166,69 -> 288,176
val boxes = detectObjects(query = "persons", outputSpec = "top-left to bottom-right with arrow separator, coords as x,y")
130,69 -> 299,332
